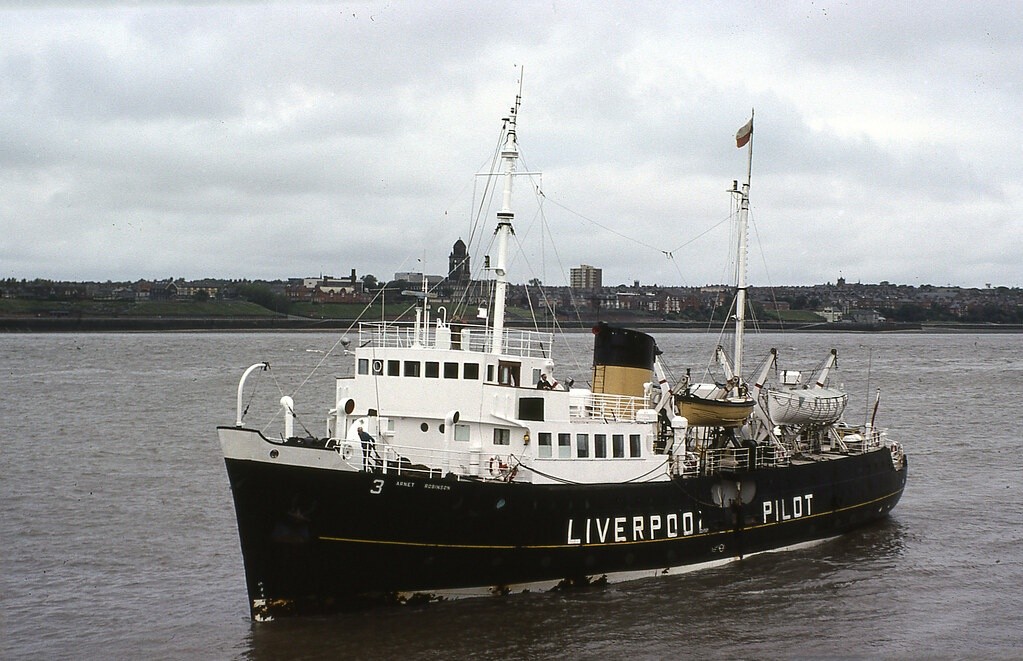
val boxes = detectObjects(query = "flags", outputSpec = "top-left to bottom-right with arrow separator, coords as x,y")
736,117 -> 753,149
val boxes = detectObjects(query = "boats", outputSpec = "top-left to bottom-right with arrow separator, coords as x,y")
215,59 -> 909,607
765,385 -> 849,426
669,391 -> 756,427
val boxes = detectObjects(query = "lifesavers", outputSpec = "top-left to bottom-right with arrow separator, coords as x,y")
489,455 -> 502,477
872,430 -> 880,447
373,361 -> 382,372
890,443 -> 898,457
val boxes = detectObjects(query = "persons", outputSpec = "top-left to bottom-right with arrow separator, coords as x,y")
358,427 -> 378,473
537,373 -> 560,391
